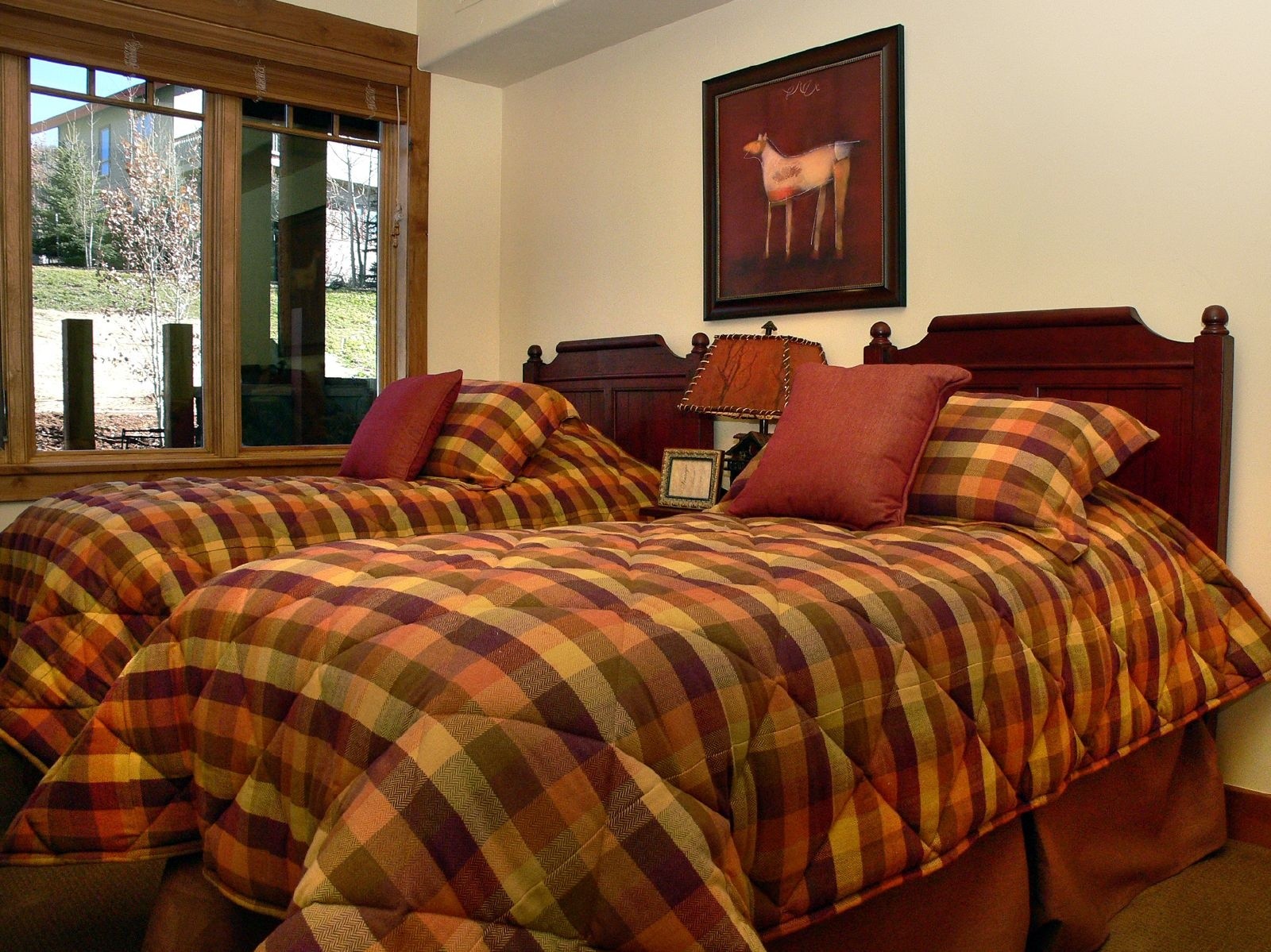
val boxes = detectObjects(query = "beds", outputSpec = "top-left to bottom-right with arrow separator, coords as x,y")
0,303 -> 1271,952
0,332 -> 712,781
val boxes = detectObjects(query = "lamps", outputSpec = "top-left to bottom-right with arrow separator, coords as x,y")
677,320 -> 829,435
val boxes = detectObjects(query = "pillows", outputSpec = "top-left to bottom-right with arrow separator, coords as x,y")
722,363 -> 973,527
335,368 -> 464,483
701,390 -> 1160,566
420,379 -> 583,489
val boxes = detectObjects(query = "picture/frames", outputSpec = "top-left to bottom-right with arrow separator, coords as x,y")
658,447 -> 722,509
700,23 -> 907,321
